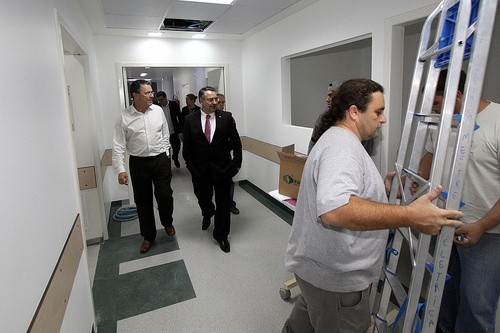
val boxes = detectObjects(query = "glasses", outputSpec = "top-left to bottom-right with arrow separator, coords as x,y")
201,97 -> 219,102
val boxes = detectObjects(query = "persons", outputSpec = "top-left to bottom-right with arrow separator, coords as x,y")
156,91 -> 181,168
282,78 -> 466,333
112,81 -> 176,254
307,80 -> 373,157
217,94 -> 241,214
416,69 -> 500,333
181,94 -> 202,141
182,86 -> 242,253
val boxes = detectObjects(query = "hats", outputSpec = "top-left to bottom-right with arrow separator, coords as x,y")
328,80 -> 346,91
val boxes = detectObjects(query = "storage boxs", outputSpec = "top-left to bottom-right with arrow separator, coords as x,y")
277,144 -> 307,200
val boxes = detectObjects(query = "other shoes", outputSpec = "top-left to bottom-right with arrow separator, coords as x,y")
172,155 -> 180,168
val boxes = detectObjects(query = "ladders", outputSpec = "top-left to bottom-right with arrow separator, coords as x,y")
368,0 -> 500,333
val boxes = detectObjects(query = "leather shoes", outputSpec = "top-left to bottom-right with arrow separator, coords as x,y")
165,224 -> 175,235
140,239 -> 154,253
231,207 -> 239,215
202,211 -> 214,230
213,231 -> 231,253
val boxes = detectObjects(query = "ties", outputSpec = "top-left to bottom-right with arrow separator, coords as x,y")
205,115 -> 211,143
